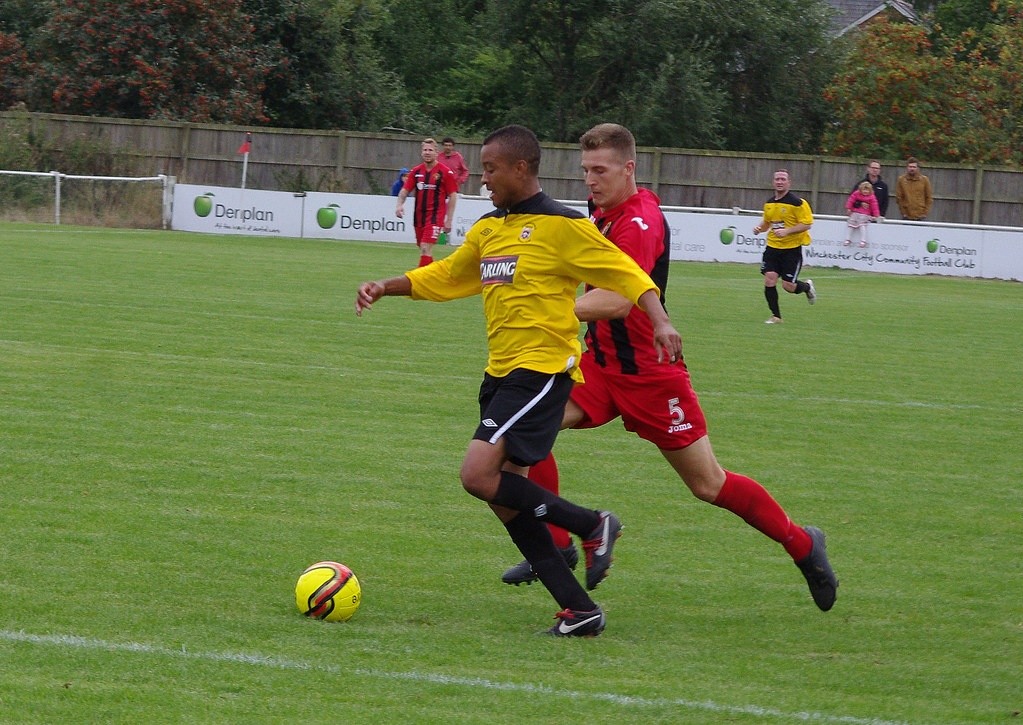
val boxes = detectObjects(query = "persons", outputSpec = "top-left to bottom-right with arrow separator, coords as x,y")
436,136 -> 470,198
844,182 -> 880,247
394,138 -> 457,268
353,125 -> 684,638
500,124 -> 840,614
752,169 -> 816,325
390,167 -> 412,197
850,161 -> 889,223
895,157 -> 933,226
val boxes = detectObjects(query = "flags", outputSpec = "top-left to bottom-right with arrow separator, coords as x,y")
236,133 -> 256,154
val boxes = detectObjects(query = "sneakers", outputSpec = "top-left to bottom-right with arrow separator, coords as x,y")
794,526 -> 839,613
765,316 -> 783,324
805,278 -> 817,305
542,604 -> 605,638
502,537 -> 579,587
583,509 -> 625,590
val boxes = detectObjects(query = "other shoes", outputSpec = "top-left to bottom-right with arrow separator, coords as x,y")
860,240 -> 866,247
843,240 -> 852,246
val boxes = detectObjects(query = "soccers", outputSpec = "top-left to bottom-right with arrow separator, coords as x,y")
294,560 -> 362,624
